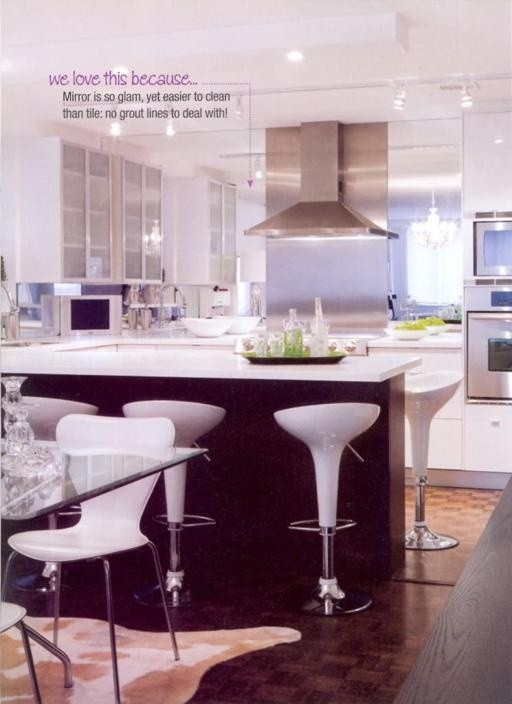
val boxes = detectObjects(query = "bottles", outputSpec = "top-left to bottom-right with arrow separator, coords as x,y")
285,296 -> 329,356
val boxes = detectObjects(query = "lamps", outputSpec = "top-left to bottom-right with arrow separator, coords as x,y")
390,76 -> 485,111
140,212 -> 165,262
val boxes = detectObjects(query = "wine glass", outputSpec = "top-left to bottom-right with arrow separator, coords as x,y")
1,371 -> 51,476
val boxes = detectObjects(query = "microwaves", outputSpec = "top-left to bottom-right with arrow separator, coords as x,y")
41,293 -> 123,337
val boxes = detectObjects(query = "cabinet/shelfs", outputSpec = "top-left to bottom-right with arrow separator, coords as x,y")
115,152 -> 164,289
14,139 -> 111,285
176,170 -> 240,291
465,404 -> 510,475
366,348 -> 465,470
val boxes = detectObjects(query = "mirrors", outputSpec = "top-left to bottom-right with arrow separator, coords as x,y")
109,131 -> 267,316
393,120 -> 465,319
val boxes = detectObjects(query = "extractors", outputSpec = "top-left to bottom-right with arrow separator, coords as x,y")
246,119 -> 400,240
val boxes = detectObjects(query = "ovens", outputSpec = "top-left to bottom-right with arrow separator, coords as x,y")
461,214 -> 512,405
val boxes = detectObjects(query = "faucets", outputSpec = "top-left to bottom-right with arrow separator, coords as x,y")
1,280 -> 20,314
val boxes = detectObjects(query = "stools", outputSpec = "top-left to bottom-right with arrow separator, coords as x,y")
406,369 -> 466,552
273,401 -> 381,616
118,400 -> 228,610
1,394 -> 102,603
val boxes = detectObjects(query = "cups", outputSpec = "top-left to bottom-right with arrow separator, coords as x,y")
1,458 -> 68,521
253,329 -> 282,357
126,308 -> 153,331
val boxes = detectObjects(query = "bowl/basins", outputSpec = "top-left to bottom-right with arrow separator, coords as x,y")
178,313 -> 262,337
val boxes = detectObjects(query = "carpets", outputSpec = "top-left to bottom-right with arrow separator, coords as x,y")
0,610 -> 303,704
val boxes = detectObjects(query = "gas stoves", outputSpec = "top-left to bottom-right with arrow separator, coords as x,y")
236,326 -> 386,356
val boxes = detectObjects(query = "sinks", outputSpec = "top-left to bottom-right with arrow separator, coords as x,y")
0,340 -> 62,348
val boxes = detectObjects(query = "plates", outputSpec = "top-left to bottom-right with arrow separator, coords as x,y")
383,324 -> 449,342
